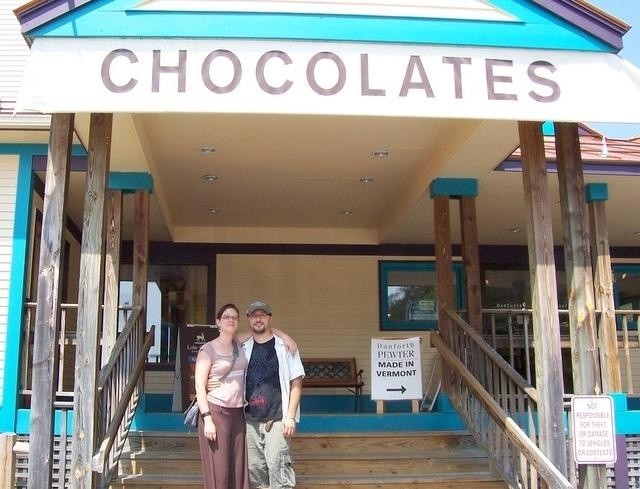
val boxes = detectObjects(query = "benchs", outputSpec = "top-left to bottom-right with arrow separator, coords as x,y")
301,357 -> 365,414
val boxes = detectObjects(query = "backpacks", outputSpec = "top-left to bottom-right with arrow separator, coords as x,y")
182,394 -> 200,428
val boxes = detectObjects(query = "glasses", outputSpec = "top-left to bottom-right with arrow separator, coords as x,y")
250,314 -> 268,320
221,316 -> 240,321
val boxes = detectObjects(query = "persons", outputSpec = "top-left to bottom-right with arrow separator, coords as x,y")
194,303 -> 298,489
203,301 -> 306,489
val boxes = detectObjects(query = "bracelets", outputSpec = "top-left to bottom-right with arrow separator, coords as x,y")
201,411 -> 211,418
285,415 -> 296,422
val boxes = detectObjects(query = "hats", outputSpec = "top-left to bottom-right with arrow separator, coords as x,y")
247,302 -> 272,316
